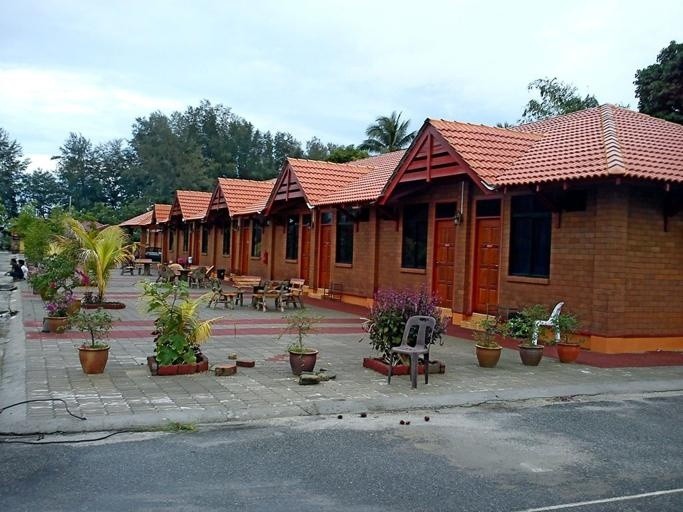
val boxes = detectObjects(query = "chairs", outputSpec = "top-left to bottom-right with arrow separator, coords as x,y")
117,255 -> 306,314
324,280 -> 345,304
531,300 -> 566,346
386,315 -> 436,389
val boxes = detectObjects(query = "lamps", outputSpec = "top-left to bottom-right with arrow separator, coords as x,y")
451,209 -> 463,226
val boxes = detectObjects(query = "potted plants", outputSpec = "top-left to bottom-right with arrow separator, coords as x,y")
500,302 -> 551,366
271,307 -> 326,377
2,201 -> 82,332
469,313 -> 508,369
55,309 -> 121,373
547,307 -> 586,363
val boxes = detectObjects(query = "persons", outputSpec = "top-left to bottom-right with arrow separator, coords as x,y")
17,259 -> 28,280
3,259 -> 22,283
